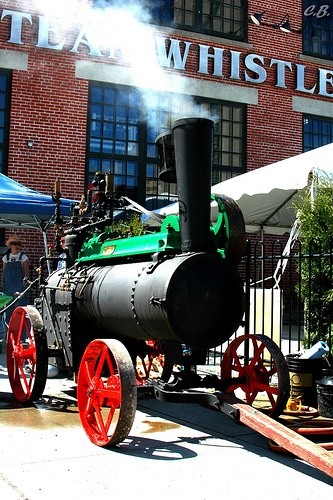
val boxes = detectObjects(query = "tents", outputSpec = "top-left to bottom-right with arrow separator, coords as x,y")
0,173 -> 118,276
57,192 -> 178,237
159,142 -> 333,290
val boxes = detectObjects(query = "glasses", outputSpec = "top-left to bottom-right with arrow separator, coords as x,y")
9,242 -> 20,246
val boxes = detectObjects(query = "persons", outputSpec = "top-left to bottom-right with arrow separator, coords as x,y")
0,235 -> 29,341
57,234 -> 67,270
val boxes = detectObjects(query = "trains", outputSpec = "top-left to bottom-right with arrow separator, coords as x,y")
4,116 -> 289,447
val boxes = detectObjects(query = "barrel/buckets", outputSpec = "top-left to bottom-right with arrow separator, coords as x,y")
315,380 -> 333,419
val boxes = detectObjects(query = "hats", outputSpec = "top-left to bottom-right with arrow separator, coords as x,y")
5,236 -> 24,247
